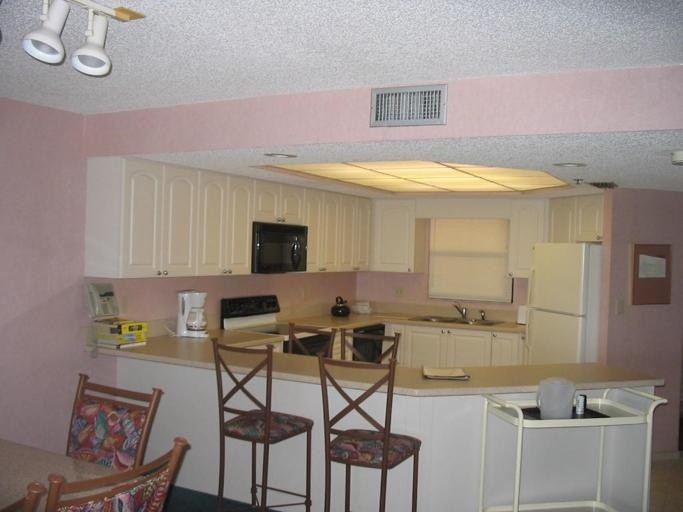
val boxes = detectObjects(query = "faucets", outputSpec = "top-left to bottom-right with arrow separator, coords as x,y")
452,303 -> 463,317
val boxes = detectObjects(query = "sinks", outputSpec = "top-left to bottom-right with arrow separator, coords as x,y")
409,315 -> 457,323
446,318 -> 505,326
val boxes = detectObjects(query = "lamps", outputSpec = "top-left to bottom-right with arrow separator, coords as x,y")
21,0 -> 146,78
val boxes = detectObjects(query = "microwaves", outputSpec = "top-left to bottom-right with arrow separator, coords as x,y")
251,221 -> 307,274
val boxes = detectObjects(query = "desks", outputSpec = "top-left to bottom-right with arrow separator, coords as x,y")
0,437 -> 143,512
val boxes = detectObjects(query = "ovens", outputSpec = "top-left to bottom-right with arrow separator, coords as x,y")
283,341 -> 333,362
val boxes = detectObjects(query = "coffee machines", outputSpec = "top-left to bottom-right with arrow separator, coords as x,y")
174,289 -> 209,338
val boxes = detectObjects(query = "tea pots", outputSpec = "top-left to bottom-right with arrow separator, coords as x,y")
330,296 -> 350,317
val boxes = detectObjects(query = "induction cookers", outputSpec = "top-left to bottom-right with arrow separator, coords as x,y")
232,322 -> 331,341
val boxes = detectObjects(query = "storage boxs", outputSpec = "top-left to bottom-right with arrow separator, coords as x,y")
93,317 -> 147,345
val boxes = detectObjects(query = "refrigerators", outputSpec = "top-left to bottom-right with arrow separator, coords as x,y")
523,241 -> 599,364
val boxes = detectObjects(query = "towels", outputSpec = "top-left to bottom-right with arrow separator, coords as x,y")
421,366 -> 471,381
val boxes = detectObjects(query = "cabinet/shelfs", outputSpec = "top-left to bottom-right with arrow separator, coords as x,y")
550,194 -> 605,243
405,324 -> 492,368
385,323 -> 405,366
508,199 -> 549,281
375,198 -> 427,274
491,331 -> 522,367
477,386 -> 669,511
85,155 -> 373,277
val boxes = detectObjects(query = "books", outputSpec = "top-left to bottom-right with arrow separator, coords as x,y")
421,365 -> 469,382
90,318 -> 149,348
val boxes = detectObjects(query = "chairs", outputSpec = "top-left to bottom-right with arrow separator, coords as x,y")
340,327 -> 401,366
66,372 -> 164,472
45,436 -> 188,512
288,321 -> 339,359
318,353 -> 422,512
0,479 -> 46,512
210,336 -> 313,512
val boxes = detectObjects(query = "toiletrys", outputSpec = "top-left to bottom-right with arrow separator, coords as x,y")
351,301 -> 372,315
517,306 -> 526,324
